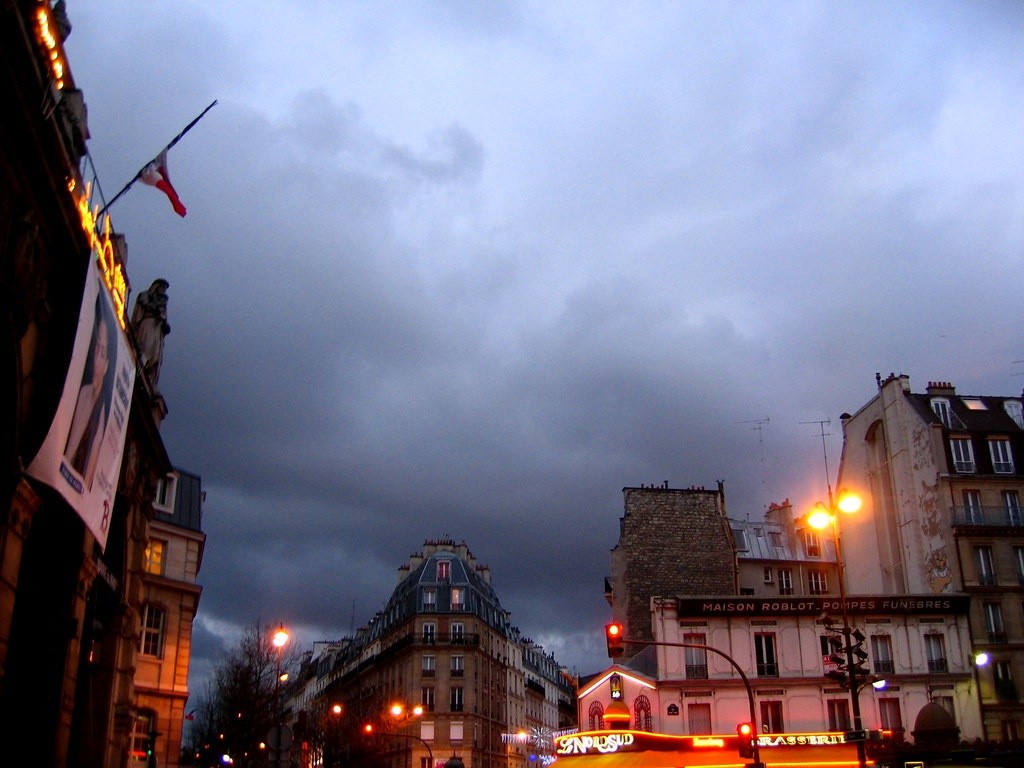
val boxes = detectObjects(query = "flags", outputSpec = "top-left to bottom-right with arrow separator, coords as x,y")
140,112 -> 201,216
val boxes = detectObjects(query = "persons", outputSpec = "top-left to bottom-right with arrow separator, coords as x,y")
129,279 -> 171,387
66,315 -> 112,491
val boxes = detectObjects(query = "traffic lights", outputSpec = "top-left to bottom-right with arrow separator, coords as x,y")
737,722 -> 754,758
605,623 -> 625,659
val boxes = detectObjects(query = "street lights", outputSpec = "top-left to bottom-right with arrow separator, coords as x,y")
807,485 -> 888,768
393,702 -> 424,767
272,622 -> 289,768
